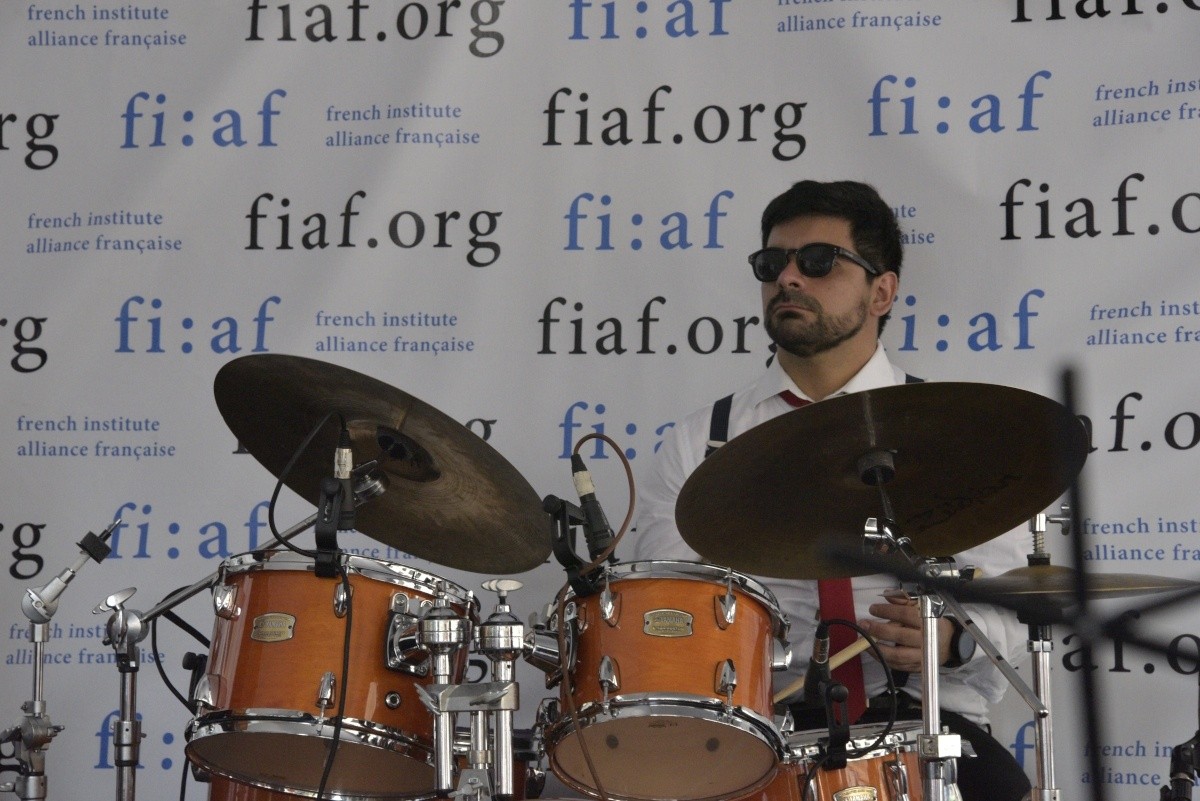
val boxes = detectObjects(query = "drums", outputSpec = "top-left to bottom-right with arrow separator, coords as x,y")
205,773 -> 333,801
544,560 -> 790,801
442,723 -> 536,801
182,548 -> 479,801
731,717 -> 961,801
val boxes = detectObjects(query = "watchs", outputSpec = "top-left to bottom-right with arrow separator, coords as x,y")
941,614 -> 976,667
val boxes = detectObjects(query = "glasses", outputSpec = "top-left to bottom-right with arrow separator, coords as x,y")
749,243 -> 881,284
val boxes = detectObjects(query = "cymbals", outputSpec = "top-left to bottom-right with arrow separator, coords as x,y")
674,384 -> 1087,579
212,355 -> 560,577
938,562 -> 1200,608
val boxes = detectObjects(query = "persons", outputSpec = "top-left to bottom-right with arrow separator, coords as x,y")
629,180 -> 1031,801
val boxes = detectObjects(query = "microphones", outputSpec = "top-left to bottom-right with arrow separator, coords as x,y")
802,622 -> 830,706
572,453 -> 614,559
331,430 -> 358,531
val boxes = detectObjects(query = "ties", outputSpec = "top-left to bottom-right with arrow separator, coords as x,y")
782,390 -> 869,726
